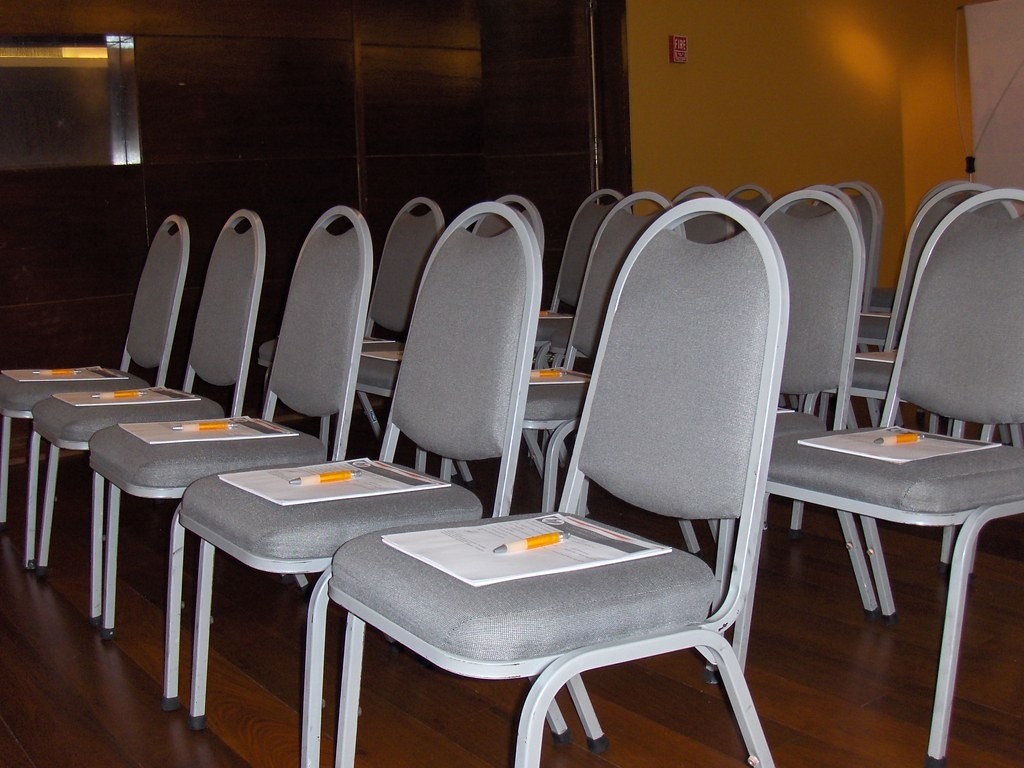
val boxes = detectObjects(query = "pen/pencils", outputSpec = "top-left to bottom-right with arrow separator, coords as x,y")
493,530 -> 571,554
874,432 -> 925,445
539,311 -> 549,317
91,391 -> 147,400
530,370 -> 568,378
397,352 -> 404,356
32,368 -> 82,375
288,470 -> 362,486
172,422 -> 240,432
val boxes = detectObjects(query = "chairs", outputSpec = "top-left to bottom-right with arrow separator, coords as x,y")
0,178 -> 1024,768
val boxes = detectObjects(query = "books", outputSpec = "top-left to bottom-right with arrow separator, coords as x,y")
0,310 -> 1004,590
382,513 -> 672,588
